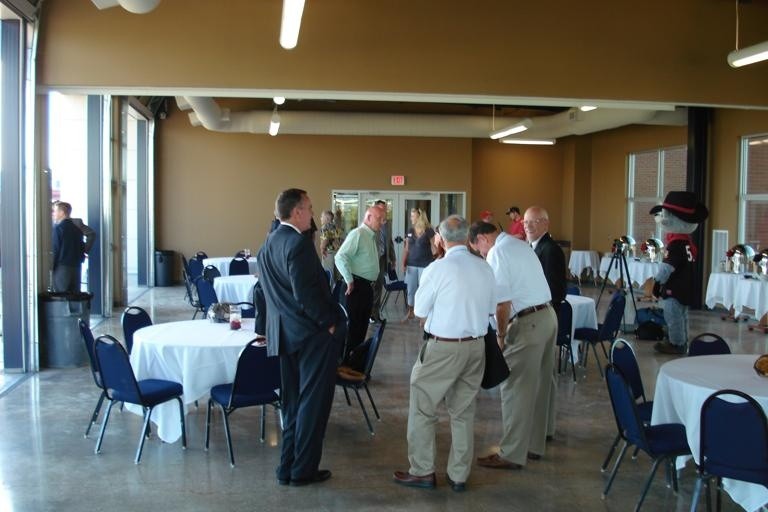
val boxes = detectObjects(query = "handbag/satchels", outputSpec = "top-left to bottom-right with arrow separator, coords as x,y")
634,307 -> 666,327
635,320 -> 668,341
481,321 -> 510,390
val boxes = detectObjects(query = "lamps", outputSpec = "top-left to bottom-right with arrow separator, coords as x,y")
488,118 -> 532,140
498,136 -> 557,146
268,97 -> 285,137
727,41 -> 767,69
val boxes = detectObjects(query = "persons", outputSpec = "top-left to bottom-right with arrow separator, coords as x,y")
480,206 -> 526,240
370,200 -> 387,322
258,186 -> 349,487
520,206 -> 567,346
335,206 -> 388,351
392,215 -> 497,490
319,207 -> 345,289
468,221 -> 558,470
402,206 -> 440,326
49,200 -> 96,292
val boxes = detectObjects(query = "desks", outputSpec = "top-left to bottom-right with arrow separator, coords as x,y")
600,254 -> 672,288
705,270 -> 768,319
569,250 -> 599,286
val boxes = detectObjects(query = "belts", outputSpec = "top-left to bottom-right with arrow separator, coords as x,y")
427,335 -> 472,342
510,303 -> 551,322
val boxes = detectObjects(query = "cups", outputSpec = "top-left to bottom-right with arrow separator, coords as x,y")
243,248 -> 250,259
228,304 -> 242,331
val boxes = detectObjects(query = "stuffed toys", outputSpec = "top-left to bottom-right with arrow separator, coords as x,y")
649,191 -> 709,353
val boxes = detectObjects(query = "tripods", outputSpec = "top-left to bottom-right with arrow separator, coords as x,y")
595,250 -> 639,334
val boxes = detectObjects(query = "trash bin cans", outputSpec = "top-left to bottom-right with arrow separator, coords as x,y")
555,240 -> 571,279
155,250 -> 174,287
41,291 -> 92,368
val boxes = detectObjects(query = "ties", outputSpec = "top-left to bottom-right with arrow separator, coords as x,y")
380,230 -> 385,256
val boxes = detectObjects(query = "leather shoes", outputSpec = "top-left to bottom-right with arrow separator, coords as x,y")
289,470 -> 332,486
477,454 -> 521,470
369,317 -> 377,324
375,318 -> 383,324
446,474 -> 465,492
392,470 -> 436,489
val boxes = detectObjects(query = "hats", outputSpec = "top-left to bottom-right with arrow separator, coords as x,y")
505,207 -> 520,215
